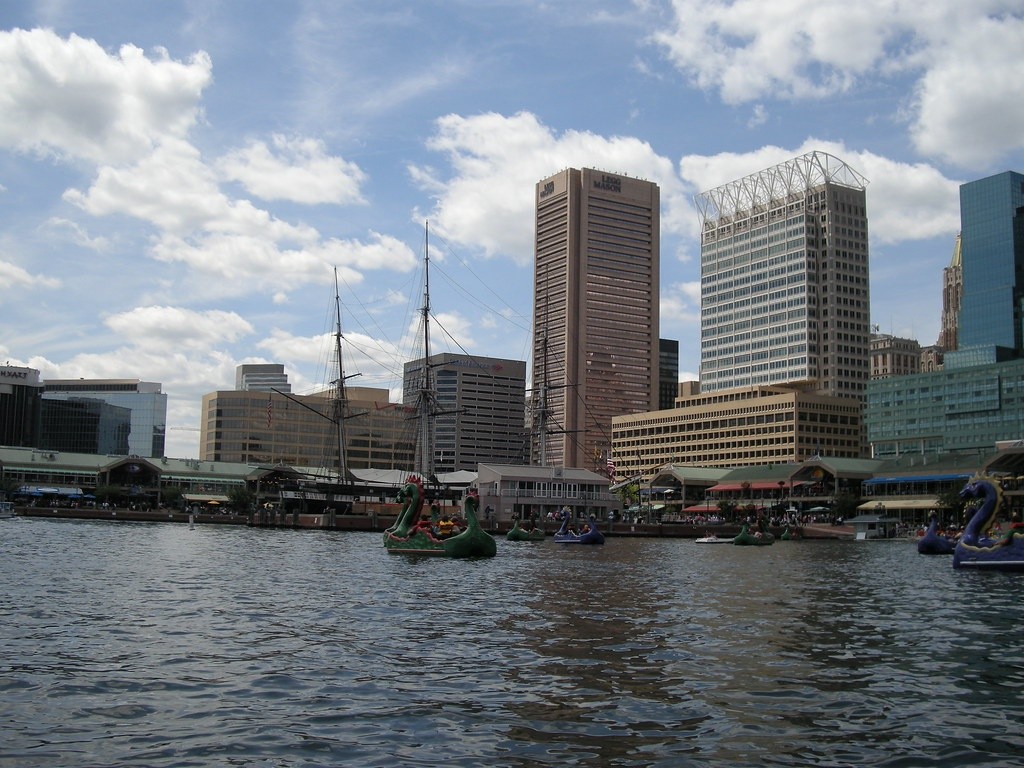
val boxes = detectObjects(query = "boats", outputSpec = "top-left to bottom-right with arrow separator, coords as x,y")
950,470 -> 1024,570
916,508 -> 960,556
780,523 -> 803,541
381,473 -> 497,559
694,530 -> 735,544
732,517 -> 775,546
552,505 -> 606,546
505,513 -> 547,542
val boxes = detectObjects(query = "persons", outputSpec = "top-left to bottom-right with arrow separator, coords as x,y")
418,515 -> 462,539
48,498 -> 118,510
185,504 -> 231,514
835,515 -> 844,526
547,510 -> 585,522
129,499 -> 156,513
769,512 -> 833,525
878,522 -> 966,538
703,531 -> 710,537
652,492 -> 679,500
618,512 -> 644,523
568,525 -> 590,536
995,514 -> 1024,547
690,513 -> 761,524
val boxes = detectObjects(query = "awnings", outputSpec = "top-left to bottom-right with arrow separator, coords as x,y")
856,500 -> 953,509
680,502 -> 780,511
182,493 -> 230,501
19,486 -> 84,498
633,488 -> 676,494
862,473 -> 982,484
629,504 -> 665,511
705,482 -> 802,491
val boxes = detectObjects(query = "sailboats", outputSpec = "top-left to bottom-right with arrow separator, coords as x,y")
245,217 -> 681,533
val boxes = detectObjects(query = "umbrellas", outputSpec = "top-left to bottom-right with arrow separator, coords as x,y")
208,500 -> 220,509
